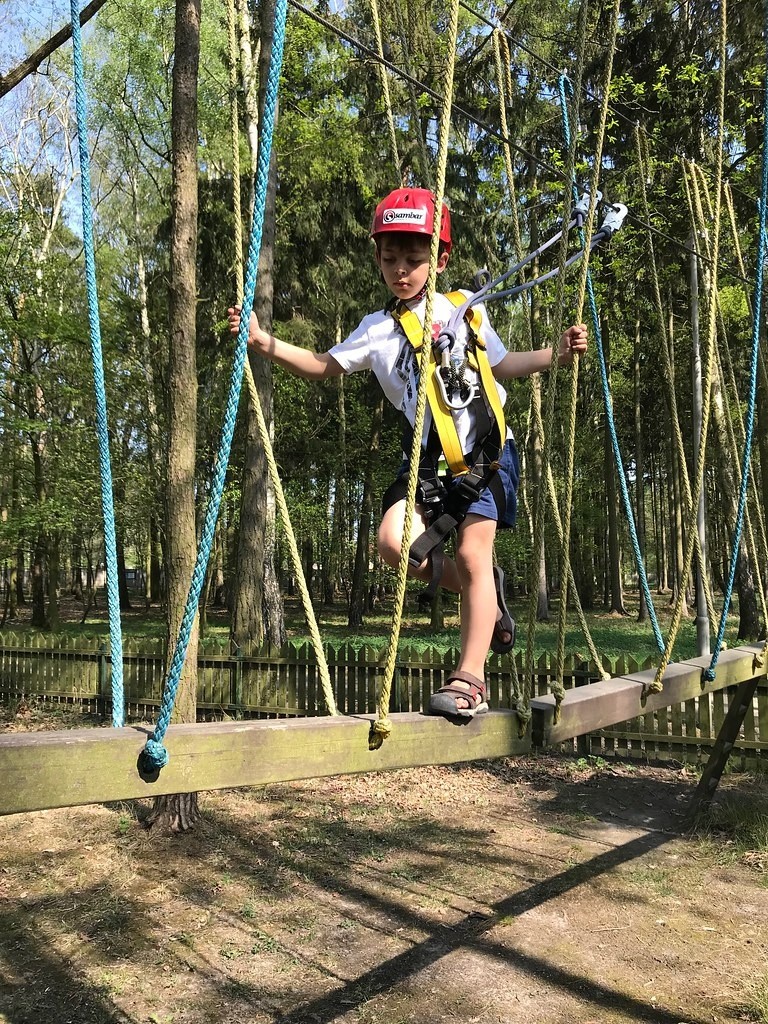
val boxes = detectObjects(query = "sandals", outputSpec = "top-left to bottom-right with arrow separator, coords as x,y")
489,567 -> 517,654
431,671 -> 491,717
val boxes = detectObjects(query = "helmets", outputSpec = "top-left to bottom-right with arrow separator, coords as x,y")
371,187 -> 453,254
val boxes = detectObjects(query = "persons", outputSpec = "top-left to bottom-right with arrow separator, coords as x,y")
226,189 -> 590,719
